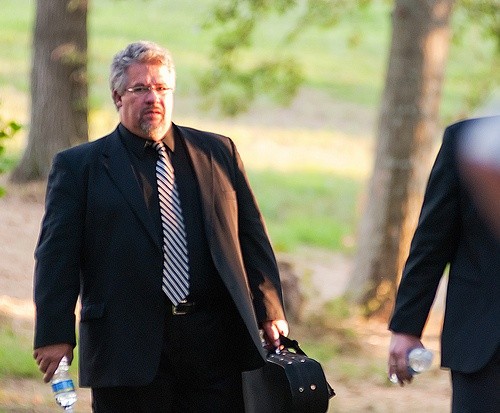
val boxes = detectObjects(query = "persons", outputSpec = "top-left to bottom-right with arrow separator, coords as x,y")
386,115 -> 500,413
33,41 -> 288,413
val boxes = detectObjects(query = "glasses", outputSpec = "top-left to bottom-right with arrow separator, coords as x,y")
125,85 -> 172,98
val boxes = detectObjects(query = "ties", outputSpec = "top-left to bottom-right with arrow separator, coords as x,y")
152,140 -> 189,306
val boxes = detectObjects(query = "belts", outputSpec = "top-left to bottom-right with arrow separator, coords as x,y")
158,293 -> 223,315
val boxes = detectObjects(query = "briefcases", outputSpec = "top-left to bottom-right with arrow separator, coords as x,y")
242,334 -> 336,413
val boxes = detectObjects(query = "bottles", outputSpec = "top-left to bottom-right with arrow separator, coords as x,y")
388,347 -> 434,383
49,355 -> 78,413
